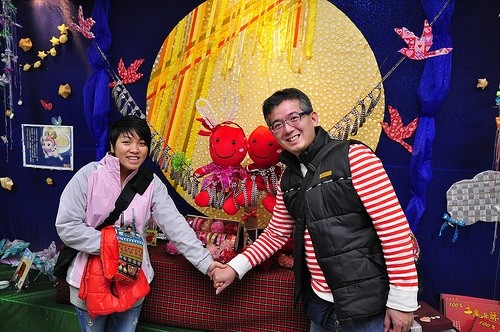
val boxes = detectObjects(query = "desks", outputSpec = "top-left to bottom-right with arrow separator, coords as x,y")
56,242 -> 310,332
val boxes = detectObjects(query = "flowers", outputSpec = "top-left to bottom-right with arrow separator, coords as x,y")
207,221 -> 238,264
165,240 -> 179,255
277,254 -> 294,269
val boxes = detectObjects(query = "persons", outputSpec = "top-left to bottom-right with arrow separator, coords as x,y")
210,88 -> 421,332
54,115 -> 227,332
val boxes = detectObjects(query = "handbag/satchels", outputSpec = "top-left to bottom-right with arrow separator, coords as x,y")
53,243 -> 79,280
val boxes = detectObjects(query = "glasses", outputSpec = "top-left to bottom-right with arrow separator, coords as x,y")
270,110 -> 308,132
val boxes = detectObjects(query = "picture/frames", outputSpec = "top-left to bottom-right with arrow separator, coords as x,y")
21,123 -> 74,172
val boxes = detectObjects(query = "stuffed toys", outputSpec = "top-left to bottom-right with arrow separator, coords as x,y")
193,93 -> 283,215
